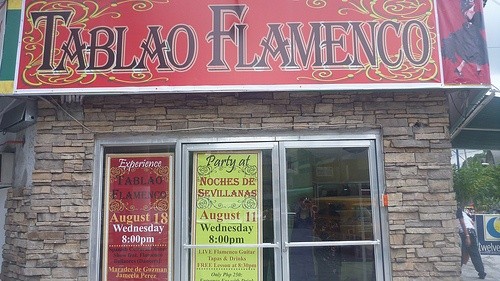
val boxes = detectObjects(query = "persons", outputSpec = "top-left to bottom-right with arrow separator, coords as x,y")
314,191 -> 341,281
289,198 -> 315,281
457,207 -> 486,279
440,0 -> 485,76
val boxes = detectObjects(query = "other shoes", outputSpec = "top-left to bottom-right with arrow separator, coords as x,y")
454,68 -> 463,77
476,70 -> 481,75
478,272 -> 486,279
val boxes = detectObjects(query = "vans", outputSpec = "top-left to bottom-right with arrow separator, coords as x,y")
310,196 -> 372,255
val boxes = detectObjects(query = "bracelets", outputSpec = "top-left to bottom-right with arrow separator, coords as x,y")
465,235 -> 470,239
